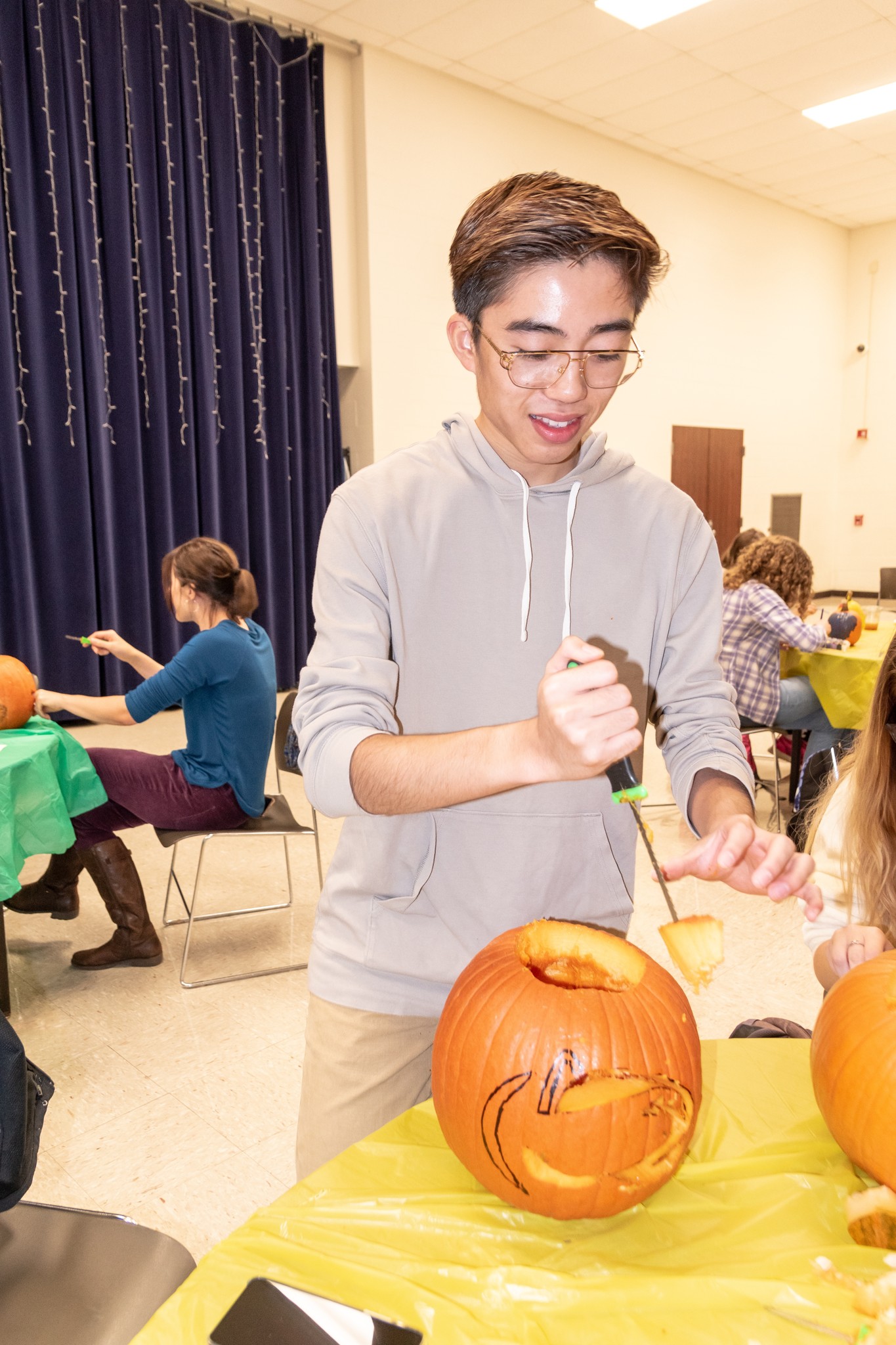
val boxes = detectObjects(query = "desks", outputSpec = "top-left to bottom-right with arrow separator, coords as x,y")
0,714 -> 108,1020
122,1035 -> 896,1345
775,602 -> 896,804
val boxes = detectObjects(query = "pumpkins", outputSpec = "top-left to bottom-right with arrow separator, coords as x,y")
827,589 -> 866,646
0,655 -> 37,730
809,949 -> 896,1193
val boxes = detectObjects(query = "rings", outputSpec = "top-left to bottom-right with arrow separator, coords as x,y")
33,702 -> 36,706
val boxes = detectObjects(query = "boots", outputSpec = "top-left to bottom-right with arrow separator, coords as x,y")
71,835 -> 161,970
4,846 -> 86,920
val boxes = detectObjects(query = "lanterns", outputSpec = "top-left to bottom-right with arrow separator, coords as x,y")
1,654 -> 39,729
434,915 -> 706,1222
825,607 -> 862,648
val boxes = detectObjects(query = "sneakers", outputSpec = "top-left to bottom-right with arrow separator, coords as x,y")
769,735 -> 806,764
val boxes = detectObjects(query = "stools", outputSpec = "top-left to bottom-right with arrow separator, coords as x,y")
738,715 -> 785,834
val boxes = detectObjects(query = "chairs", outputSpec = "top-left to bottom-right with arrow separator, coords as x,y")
153,689 -> 324,989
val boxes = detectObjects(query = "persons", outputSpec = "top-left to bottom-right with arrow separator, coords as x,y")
721,527 -> 767,575
0,534 -> 281,972
802,634 -> 896,989
268,164 -> 830,1194
719,534 -> 851,807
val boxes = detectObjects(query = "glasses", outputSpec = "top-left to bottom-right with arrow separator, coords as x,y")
473,323 -> 642,389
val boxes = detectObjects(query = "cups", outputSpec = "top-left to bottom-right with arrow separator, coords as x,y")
863,605 -> 882,631
